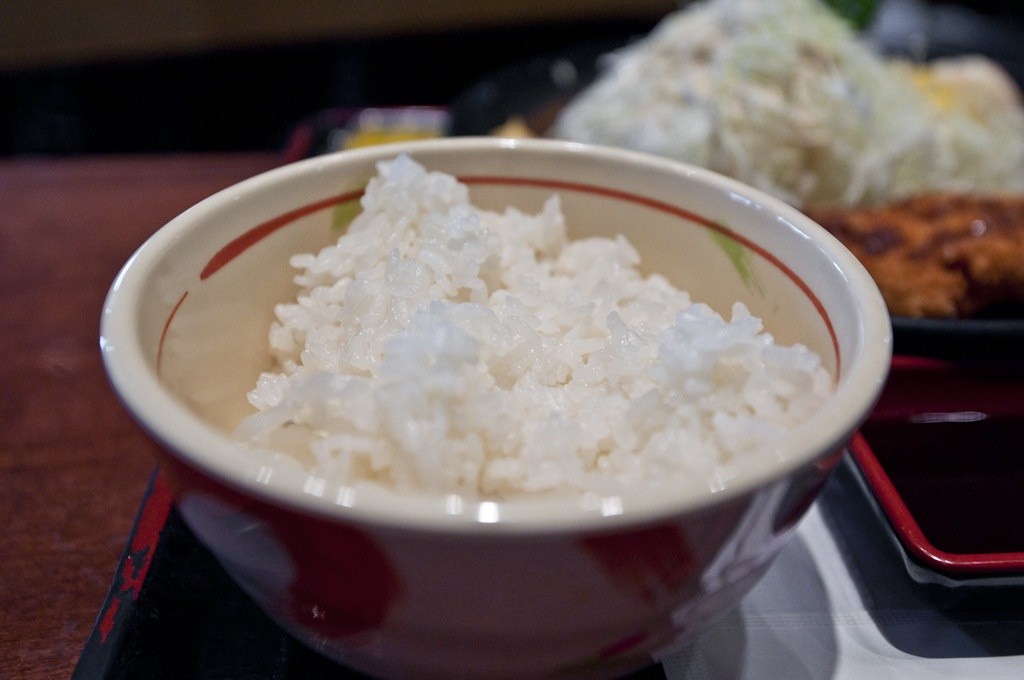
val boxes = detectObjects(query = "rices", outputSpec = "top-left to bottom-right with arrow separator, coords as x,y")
236,153 -> 835,499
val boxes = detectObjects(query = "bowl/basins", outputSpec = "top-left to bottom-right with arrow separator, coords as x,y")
98,135 -> 891,680
